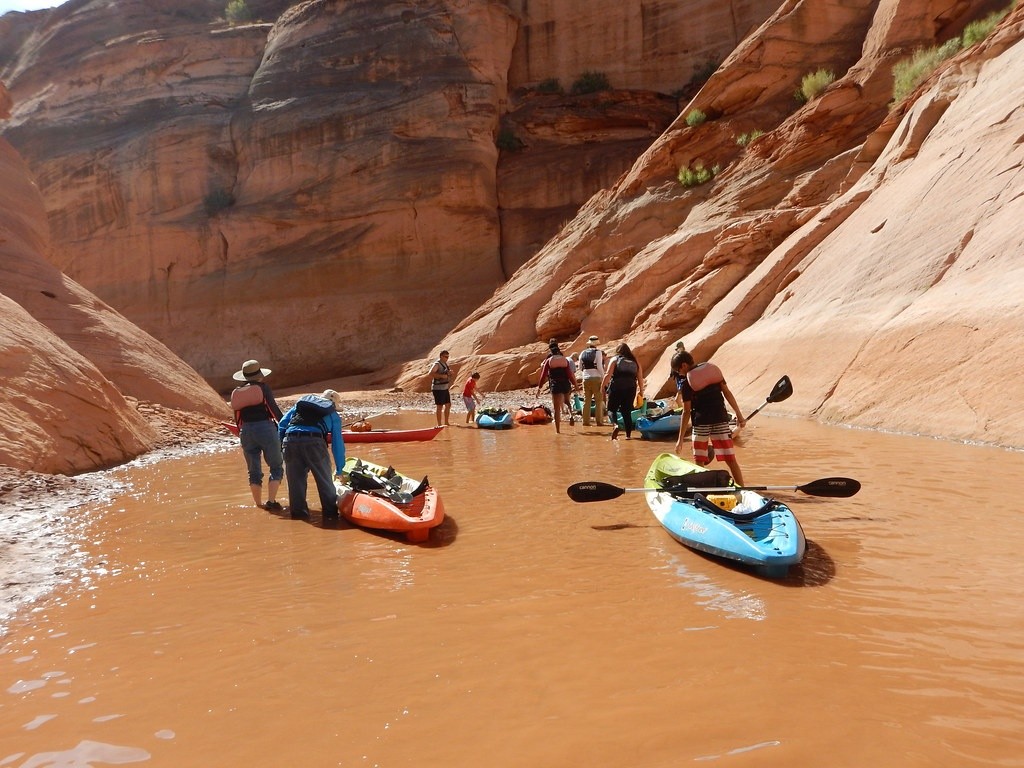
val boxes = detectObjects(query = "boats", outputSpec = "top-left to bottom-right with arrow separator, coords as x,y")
643,453 -> 806,577
515,406 -> 553,425
572,393 -> 737,439
332,457 -> 445,541
221,421 -> 445,443
475,411 -> 513,429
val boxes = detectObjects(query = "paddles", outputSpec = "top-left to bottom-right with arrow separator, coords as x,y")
352,458 -> 413,504
704,374 -> 794,467
565,476 -> 862,503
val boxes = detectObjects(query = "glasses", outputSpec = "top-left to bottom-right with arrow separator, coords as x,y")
574,356 -> 579,360
442,355 -> 449,359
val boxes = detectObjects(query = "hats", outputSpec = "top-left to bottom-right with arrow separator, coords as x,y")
232,359 -> 273,382
319,389 -> 344,412
586,335 -> 600,345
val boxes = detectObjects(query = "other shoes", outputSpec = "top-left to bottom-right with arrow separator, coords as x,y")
611,425 -> 619,439
266,499 -> 283,511
625,436 -> 631,440
570,416 -> 574,426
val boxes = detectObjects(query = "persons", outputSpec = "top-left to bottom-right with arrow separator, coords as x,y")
278,388 -> 347,524
602,351 -> 610,372
231,360 -> 284,512
536,338 -> 579,433
566,351 -> 579,378
578,335 -> 605,426
670,351 -> 747,488
463,372 -> 486,422
674,342 -> 686,408
600,343 -> 644,439
429,350 -> 455,426
